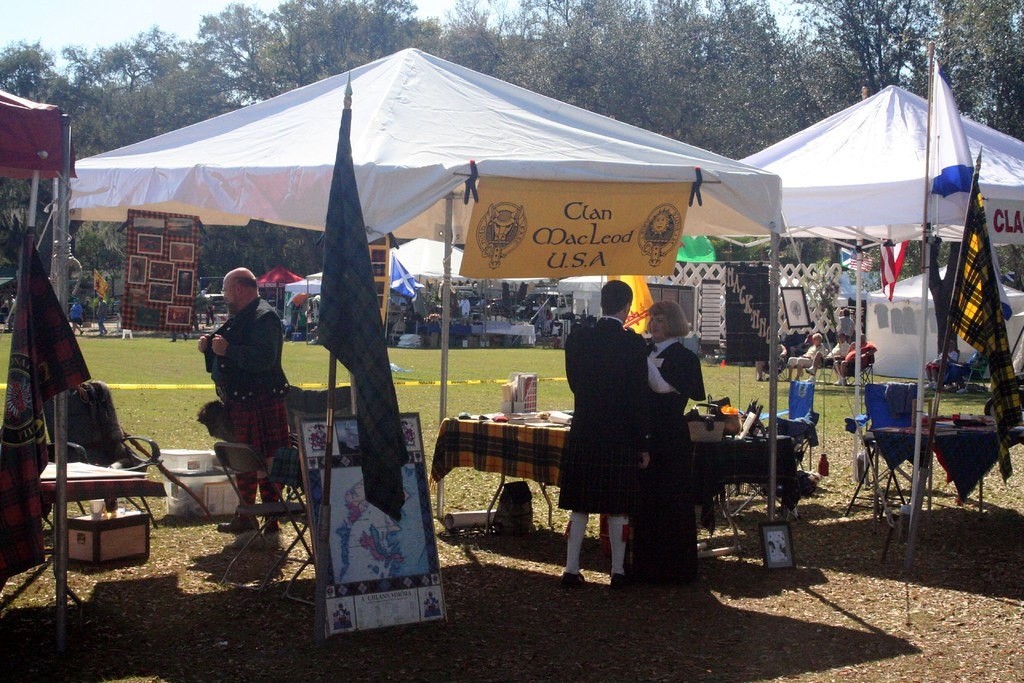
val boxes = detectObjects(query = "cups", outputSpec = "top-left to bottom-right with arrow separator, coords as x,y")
89,500 -> 104,520
118,501 -> 126,515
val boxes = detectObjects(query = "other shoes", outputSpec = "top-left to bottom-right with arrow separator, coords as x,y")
807,377 -> 815,382
562,573 -> 585,588
956,389 -> 969,393
924,383 -> 933,387
610,573 -> 638,588
932,385 -> 937,389
784,378 -> 791,382
834,379 -> 847,384
806,368 -> 815,375
217,512 -> 259,532
264,517 -> 277,533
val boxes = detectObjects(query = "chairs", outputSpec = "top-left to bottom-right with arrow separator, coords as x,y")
213,386 -> 351,607
389,322 -> 406,347
43,381 -> 166,529
115,313 -> 133,338
739,342 -> 989,526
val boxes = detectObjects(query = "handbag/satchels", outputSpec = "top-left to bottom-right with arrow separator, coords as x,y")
708,395 -> 731,420
45,382 -> 128,465
197,401 -> 234,442
489,482 -> 533,536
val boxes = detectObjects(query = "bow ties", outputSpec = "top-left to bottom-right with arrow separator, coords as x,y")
646,340 -> 658,357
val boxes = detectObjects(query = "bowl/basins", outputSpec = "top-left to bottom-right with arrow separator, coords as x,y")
161,449 -> 212,474
722,413 -> 742,437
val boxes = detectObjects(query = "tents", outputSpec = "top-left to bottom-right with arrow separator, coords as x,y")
865,263 -> 1024,380
253,234 -> 609,347
739,85 -> 1024,536
0,89 -> 74,655
52,46 -> 784,525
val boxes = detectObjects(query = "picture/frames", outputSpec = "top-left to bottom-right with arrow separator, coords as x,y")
780,286 -> 812,329
759,521 -> 797,572
127,233 -> 195,326
370,236 -> 388,313
294,412 -> 448,642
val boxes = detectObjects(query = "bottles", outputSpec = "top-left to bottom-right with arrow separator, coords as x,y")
857,451 -> 868,484
809,472 -> 822,483
819,454 -> 830,477
899,505 -> 915,544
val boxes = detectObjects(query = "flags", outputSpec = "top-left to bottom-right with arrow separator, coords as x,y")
947,175 -> 1024,483
389,254 -> 425,302
879,238 -> 910,300
316,110 -> 407,518
841,248 -> 873,271
0,227 -> 91,588
93,268 -> 109,294
930,60 -> 1012,319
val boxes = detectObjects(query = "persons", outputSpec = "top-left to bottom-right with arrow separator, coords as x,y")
457,294 -> 471,315
925,339 -> 988,394
198,266 -> 290,534
755,309 -> 877,385
96,295 -> 107,335
559,282 -> 705,595
69,298 -> 83,335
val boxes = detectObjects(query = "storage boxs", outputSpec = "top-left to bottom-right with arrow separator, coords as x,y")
67,510 -> 153,565
160,450 -> 240,516
290,332 -> 302,340
425,333 -> 500,348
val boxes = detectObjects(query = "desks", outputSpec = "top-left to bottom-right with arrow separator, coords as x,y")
421,323 -> 471,348
474,321 -> 536,348
435,412 -> 799,574
40,462 -> 167,619
872,425 -> 1024,563
